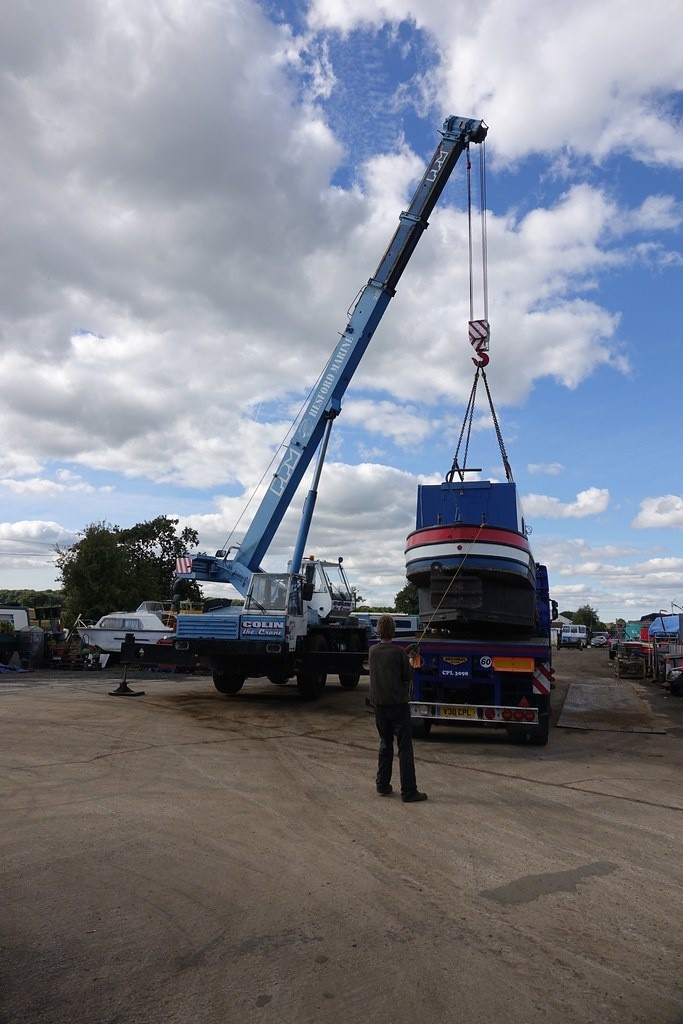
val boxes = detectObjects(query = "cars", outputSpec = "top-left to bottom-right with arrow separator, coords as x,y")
665,666 -> 683,689
590,635 -> 607,648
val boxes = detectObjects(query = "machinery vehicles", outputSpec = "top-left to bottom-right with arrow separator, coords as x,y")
107,113 -> 490,702
33,605 -> 67,657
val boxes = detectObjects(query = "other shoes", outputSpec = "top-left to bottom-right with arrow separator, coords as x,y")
401,791 -> 427,802
377,785 -> 392,795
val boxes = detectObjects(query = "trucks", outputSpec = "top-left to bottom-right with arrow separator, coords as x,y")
348,612 -> 418,674
408,561 -> 559,745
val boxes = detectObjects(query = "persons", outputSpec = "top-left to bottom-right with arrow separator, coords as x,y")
608,637 -> 611,651
368,614 -> 428,803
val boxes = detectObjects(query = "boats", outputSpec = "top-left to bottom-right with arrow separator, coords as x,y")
75,612 -> 176,652
403,509 -> 538,589
